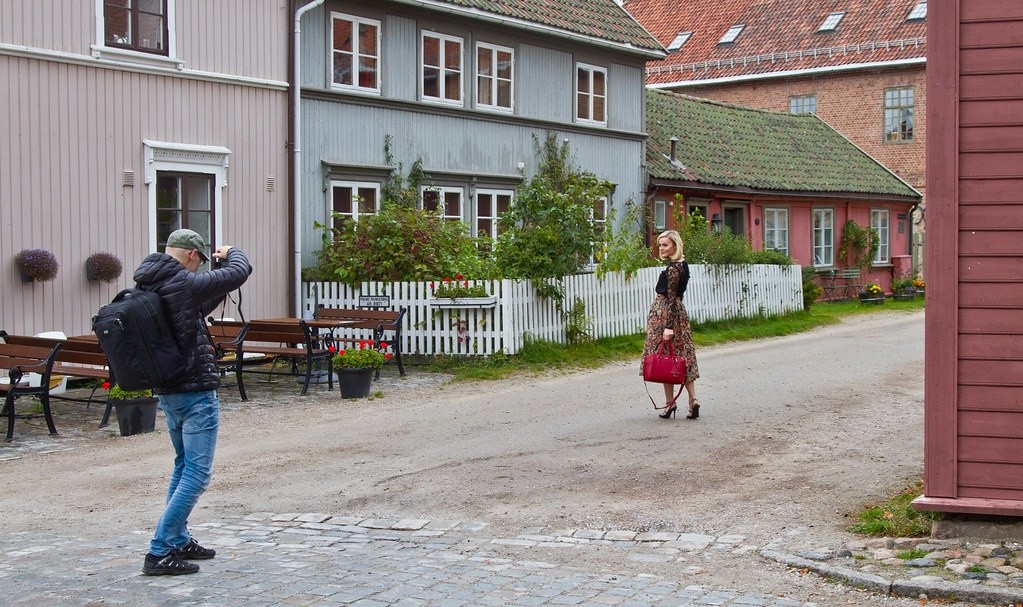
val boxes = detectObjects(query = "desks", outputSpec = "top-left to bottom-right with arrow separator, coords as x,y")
249,320 -> 364,383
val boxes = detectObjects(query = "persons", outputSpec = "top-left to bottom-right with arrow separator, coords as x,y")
133,228 -> 253,575
640,229 -> 700,420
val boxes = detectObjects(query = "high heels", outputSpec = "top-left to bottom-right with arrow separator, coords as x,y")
686,398 -> 700,419
658,400 -> 678,419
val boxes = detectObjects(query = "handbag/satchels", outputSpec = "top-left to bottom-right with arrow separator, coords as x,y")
643,337 -> 686,384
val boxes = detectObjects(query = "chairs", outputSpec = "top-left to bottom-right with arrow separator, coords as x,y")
819,267 -> 864,305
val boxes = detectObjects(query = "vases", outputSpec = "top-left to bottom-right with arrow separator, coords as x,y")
914,286 -> 925,297
87,271 -> 96,281
115,398 -> 160,435
336,368 -> 374,398
891,289 -> 914,301
20,271 -> 35,283
858,290 -> 885,305
429,295 -> 496,309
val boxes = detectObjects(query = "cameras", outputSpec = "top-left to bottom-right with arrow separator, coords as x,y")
215,257 -> 228,269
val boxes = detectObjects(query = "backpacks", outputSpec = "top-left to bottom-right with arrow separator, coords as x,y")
91,277 -> 198,391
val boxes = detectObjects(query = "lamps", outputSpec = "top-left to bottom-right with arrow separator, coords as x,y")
711,213 -> 722,237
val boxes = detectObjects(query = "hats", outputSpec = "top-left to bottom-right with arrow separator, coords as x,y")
166,230 -> 212,263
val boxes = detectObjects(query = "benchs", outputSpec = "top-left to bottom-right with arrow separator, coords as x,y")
0,342 -> 62,441
208,316 -> 336,395
289,305 -> 406,381
206,323 -> 251,401
0,329 -> 116,427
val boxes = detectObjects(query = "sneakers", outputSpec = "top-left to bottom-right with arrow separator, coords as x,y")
142,552 -> 199,576
174,538 -> 215,560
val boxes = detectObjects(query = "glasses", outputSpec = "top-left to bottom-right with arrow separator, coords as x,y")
189,250 -> 206,267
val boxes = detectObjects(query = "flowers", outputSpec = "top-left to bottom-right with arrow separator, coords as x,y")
16,249 -> 58,282
103,381 -> 152,399
327,343 -> 392,370
890,267 -> 925,289
859,278 -> 882,293
86,252 -> 124,283
431,274 -> 488,302
